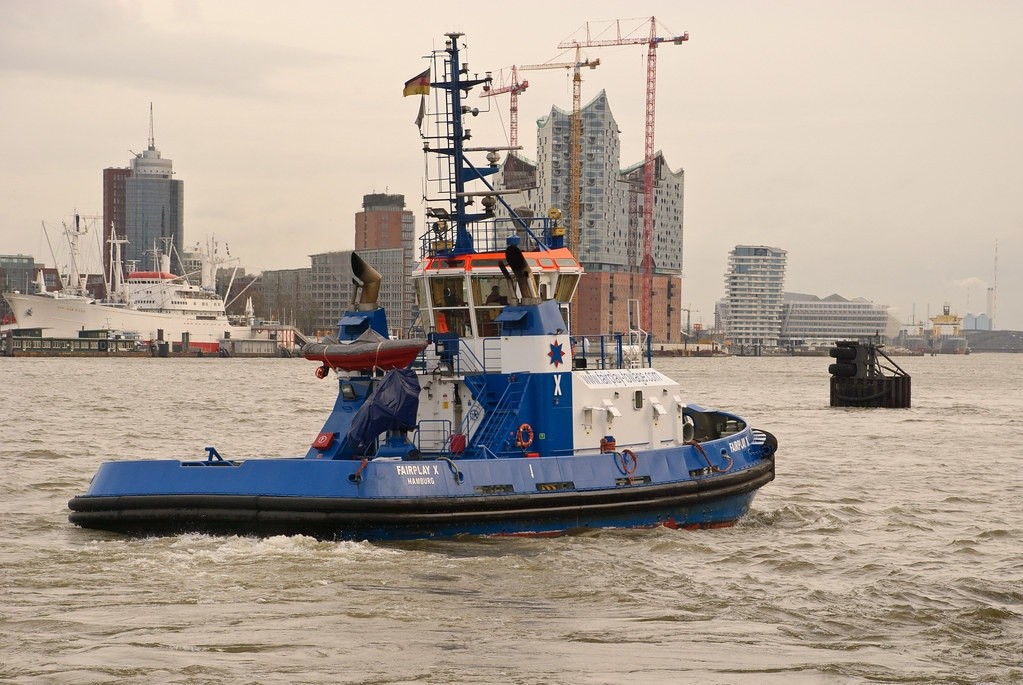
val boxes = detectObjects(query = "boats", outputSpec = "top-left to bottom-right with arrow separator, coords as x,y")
68,31 -> 777,539
302,329 -> 428,379
0,208 -> 295,352
893,303 -> 969,355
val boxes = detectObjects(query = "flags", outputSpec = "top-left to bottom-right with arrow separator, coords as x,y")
403,67 -> 430,98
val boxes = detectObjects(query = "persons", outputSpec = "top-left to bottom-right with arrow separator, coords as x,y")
485,285 -> 508,313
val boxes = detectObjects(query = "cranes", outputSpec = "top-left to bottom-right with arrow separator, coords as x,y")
557,16 -> 688,344
479,64 -> 529,189
518,44 -> 602,342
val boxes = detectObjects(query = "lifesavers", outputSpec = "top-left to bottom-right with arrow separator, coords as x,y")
518,423 -> 534,447
